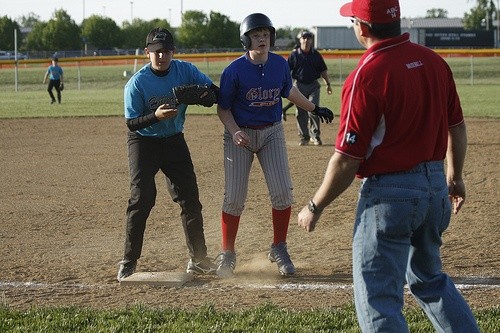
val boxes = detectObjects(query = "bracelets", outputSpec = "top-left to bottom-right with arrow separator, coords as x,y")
235,131 -> 242,134
327,83 -> 330,85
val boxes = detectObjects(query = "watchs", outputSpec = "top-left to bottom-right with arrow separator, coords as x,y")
308,200 -> 323,214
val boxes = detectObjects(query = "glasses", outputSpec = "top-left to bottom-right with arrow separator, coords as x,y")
350,16 -> 371,28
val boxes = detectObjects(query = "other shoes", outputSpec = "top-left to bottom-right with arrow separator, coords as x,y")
50,99 -> 56,105
298,139 -> 308,146
282,108 -> 287,122
310,137 -> 322,146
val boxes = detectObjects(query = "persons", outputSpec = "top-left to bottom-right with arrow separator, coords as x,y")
44,58 -> 64,104
117,29 -> 220,280
288,33 -> 332,145
299,0 -> 479,333
217,14 -> 334,275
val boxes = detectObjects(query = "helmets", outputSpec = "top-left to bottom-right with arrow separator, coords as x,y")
240,13 -> 276,51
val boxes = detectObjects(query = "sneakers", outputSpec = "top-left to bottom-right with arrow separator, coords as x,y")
116,258 -> 136,282
187,258 -> 219,275
216,250 -> 236,278
267,242 -> 294,276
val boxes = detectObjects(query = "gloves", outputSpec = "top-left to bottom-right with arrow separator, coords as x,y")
310,105 -> 334,123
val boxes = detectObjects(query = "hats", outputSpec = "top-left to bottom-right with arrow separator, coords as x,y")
146,27 -> 174,52
297,30 -> 313,38
340,0 -> 401,24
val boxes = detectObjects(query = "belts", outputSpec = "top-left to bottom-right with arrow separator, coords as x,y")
242,122 -> 273,130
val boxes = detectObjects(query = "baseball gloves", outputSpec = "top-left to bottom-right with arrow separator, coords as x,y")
59,82 -> 64,91
171,83 -> 217,109
